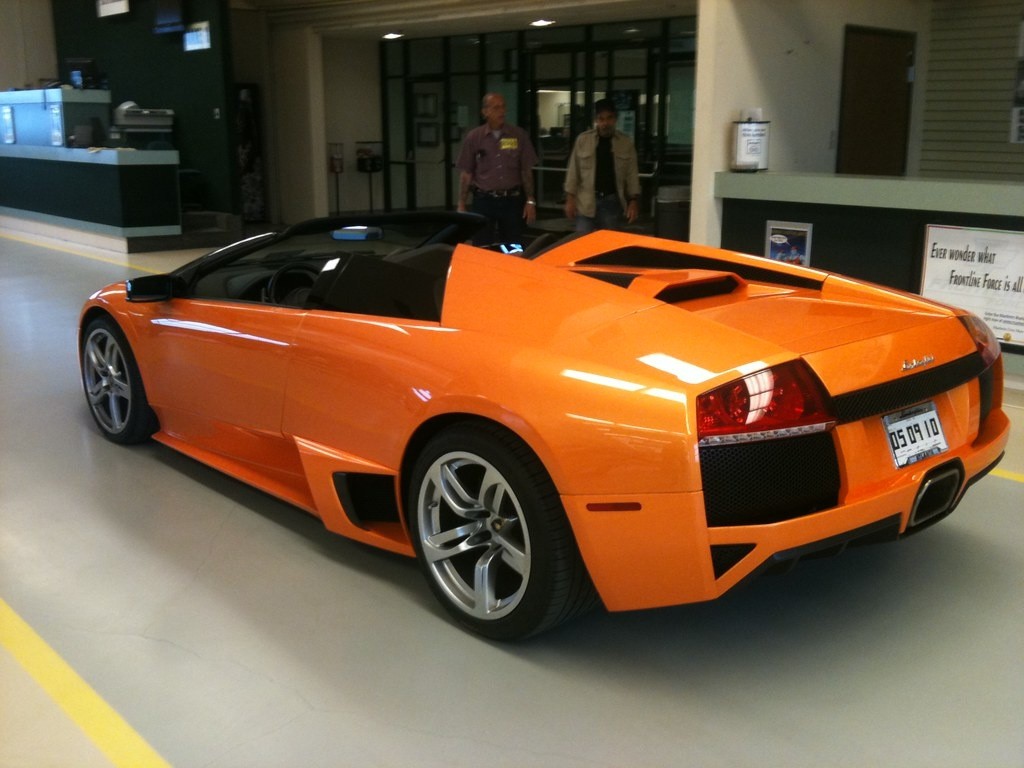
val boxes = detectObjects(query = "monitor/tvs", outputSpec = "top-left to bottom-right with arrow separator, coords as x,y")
58,58 -> 100,90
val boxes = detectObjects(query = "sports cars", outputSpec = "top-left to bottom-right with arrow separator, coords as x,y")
76,208 -> 1013,645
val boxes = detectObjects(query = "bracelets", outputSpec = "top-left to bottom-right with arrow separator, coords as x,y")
629,198 -> 639,201
525,192 -> 534,196
527,200 -> 536,205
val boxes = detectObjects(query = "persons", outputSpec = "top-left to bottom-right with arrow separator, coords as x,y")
452,93 -> 540,226
563,99 -> 640,233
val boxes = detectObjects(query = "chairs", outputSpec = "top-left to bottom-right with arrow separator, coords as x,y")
300,230 -> 580,322
